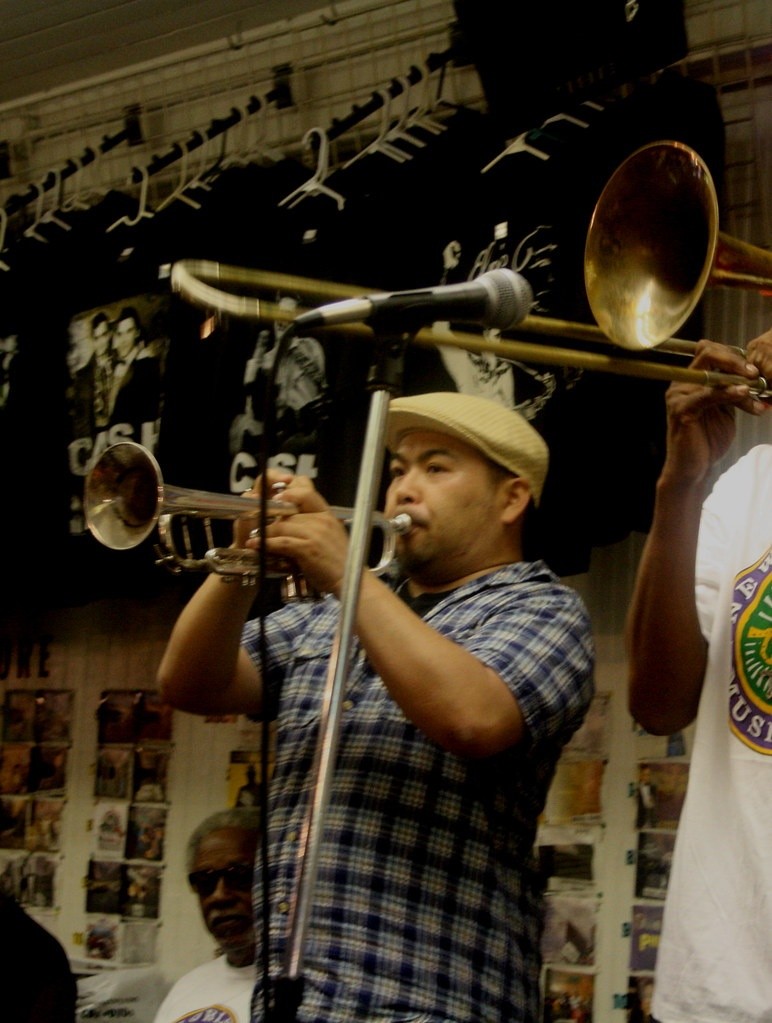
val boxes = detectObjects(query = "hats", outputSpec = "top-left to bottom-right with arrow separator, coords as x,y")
383,392 -> 547,506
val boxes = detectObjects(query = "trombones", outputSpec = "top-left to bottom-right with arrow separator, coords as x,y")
171,138 -> 772,399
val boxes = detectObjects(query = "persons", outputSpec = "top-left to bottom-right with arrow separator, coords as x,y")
225,296 -> 325,454
152,391 -> 596,1023
152,809 -> 264,1023
624,328 -> 772,1023
64,306 -> 151,535
0,893 -> 79,1023
0,331 -> 20,407
431,239 -> 515,410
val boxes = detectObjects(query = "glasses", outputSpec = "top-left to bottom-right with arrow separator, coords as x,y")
184,858 -> 257,899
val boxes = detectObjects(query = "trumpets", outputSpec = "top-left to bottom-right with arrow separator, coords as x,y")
84,440 -> 413,607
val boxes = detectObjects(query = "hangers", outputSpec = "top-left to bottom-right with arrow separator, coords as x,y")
0,51 -> 603,275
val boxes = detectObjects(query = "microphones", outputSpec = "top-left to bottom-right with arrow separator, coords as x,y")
297,268 -> 534,329
